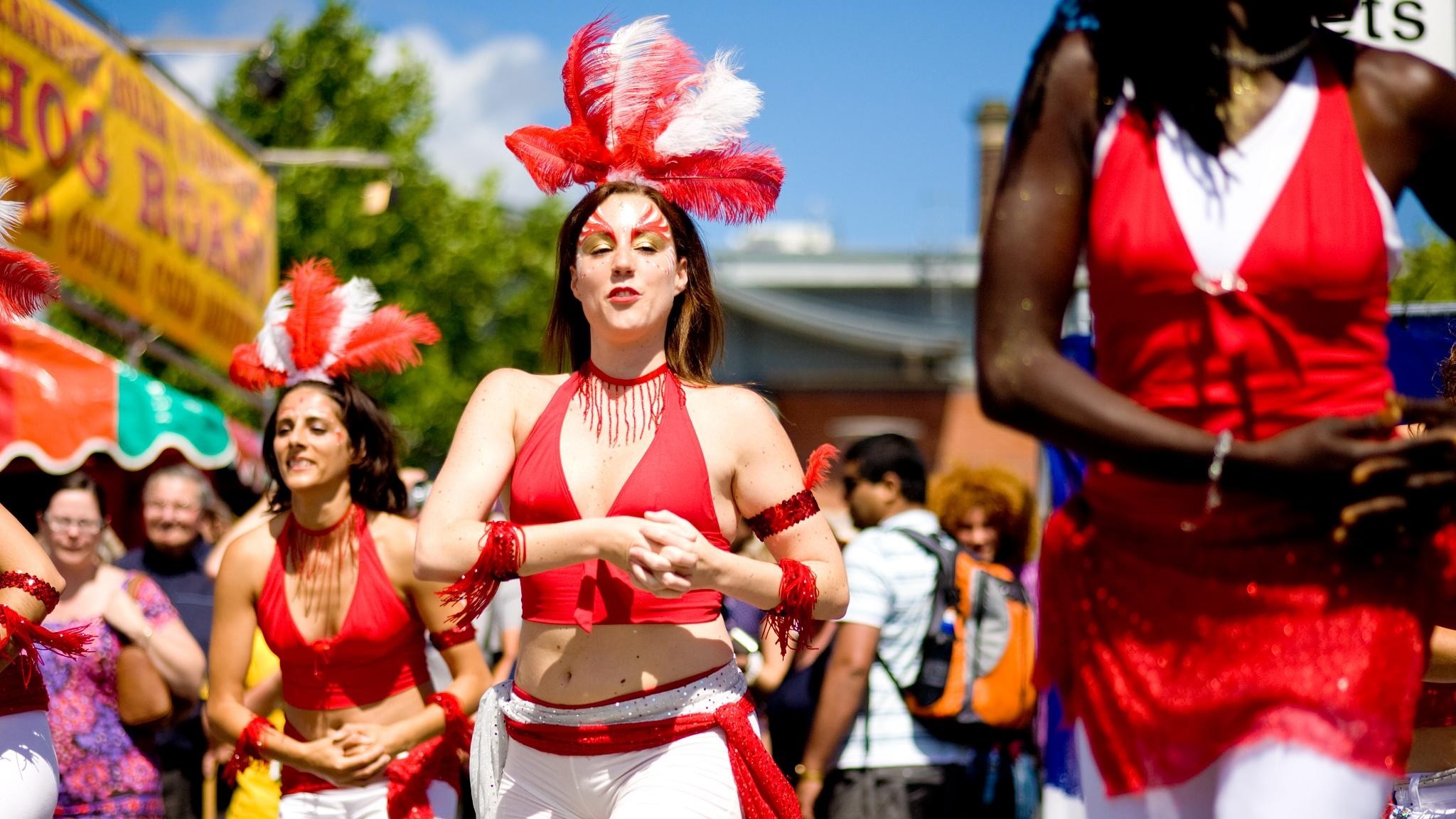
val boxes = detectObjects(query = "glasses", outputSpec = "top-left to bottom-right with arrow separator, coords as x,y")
43,516 -> 110,537
143,496 -> 202,511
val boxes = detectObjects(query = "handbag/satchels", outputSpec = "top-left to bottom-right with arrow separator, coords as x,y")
115,573 -> 174,727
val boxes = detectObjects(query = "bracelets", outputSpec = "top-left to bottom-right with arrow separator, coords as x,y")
795,763 -> 824,785
1207,430 -> 1234,478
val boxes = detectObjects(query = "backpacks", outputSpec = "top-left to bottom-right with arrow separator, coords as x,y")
867,524 -> 1043,749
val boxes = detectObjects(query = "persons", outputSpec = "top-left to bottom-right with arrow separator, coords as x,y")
1,456 -> 290,819
197,376 -> 491,819
409,9 -> 853,819
717,431 -> 1043,819
395,460 -> 525,819
966,1 -> 1455,819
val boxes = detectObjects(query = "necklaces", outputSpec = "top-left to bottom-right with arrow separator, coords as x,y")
1216,33 -> 1313,69
287,500 -> 356,617
572,356 -> 711,447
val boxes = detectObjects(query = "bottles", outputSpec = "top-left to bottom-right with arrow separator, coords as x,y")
919,605 -> 958,704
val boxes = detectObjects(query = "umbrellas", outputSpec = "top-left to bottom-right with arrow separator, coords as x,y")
0,305 -> 262,485
